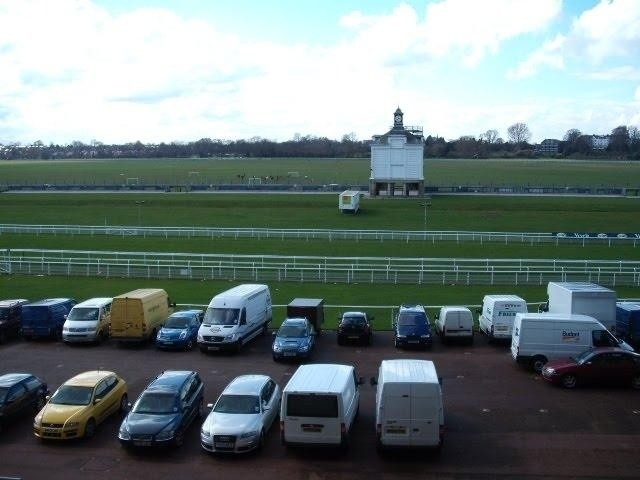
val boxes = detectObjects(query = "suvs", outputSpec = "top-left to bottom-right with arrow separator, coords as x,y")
112,369 -> 206,453
0,297 -> 26,338
393,303 -> 433,352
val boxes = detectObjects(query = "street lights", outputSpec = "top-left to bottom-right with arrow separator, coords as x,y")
416,201 -> 434,241
135,200 -> 145,226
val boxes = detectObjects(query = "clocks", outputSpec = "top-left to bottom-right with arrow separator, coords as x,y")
396,114 -> 402,123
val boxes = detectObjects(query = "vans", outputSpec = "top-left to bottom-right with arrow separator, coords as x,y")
276,362 -> 366,449
61,297 -> 113,346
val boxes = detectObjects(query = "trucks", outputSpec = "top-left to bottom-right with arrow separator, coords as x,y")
369,356 -> 450,452
433,281 -> 640,371
196,280 -> 274,358
108,285 -> 177,343
20,296 -> 77,341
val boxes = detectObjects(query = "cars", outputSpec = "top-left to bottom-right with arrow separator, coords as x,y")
0,370 -> 50,430
197,370 -> 283,456
30,366 -> 130,444
541,346 -> 640,392
154,306 -> 204,355
334,308 -> 375,349
264,315 -> 316,360
